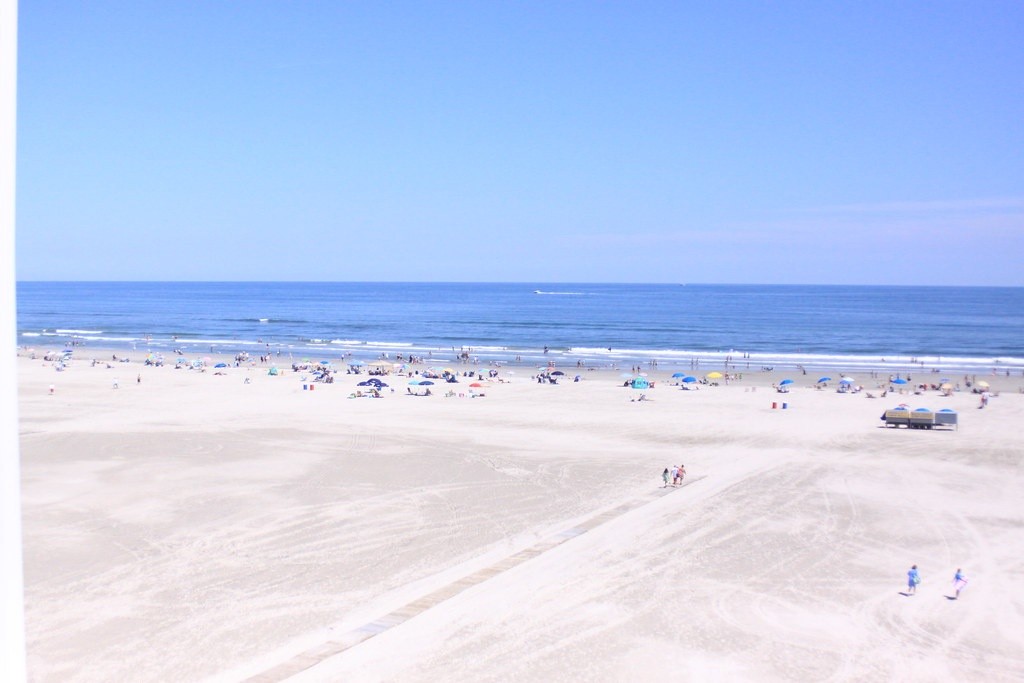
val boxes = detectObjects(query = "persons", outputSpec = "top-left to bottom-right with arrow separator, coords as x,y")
661,464 -> 687,489
907,565 -> 918,595
954,569 -> 968,599
526,341 -> 1019,412
19,331 -> 516,409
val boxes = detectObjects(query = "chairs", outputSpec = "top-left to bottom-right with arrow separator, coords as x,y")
934,412 -> 959,430
886,408 -> 909,428
910,412 -> 935,429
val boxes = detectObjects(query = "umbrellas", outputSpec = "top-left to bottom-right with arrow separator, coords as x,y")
682,376 -> 697,384
839,377 -> 855,388
818,377 -> 831,386
672,373 -> 685,379
551,371 -> 564,378
348,360 -> 369,371
780,380 -> 793,389
367,360 -> 392,372
707,372 -> 722,384
